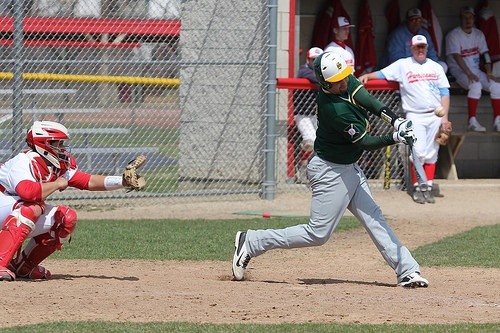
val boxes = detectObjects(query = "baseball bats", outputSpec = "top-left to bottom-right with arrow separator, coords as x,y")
407,137 -> 430,193
383,145 -> 390,190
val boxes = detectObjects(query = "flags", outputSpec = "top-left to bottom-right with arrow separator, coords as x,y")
311,0 -> 377,79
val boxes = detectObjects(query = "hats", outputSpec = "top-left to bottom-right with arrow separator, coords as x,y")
410,34 -> 427,47
331,16 -> 355,30
460,5 -> 475,16
307,47 -> 324,59
406,7 -> 423,19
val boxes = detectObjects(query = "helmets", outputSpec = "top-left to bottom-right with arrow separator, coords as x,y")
26,120 -> 71,170
313,51 -> 353,88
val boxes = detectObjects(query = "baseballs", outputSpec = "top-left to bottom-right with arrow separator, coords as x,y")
434,105 -> 446,118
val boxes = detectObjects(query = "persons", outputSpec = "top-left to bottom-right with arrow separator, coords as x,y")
383,8 -> 448,73
358,35 -> 452,204
324,16 -> 355,76
446,4 -> 500,132
0,121 -> 147,281
232,52 -> 429,287
293,47 -> 324,182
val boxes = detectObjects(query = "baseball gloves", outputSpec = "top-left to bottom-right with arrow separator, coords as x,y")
434,121 -> 452,145
122,154 -> 147,193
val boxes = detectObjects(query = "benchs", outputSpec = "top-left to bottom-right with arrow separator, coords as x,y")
0,88 -> 158,171
447,132 -> 500,178
449,77 -> 490,96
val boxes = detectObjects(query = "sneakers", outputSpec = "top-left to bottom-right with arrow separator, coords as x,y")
468,116 -> 485,132
397,272 -> 429,288
232,231 -> 251,281
493,115 -> 500,131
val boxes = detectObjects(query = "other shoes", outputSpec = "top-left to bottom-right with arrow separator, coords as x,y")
0,267 -> 16,281
423,191 -> 436,204
9,259 -> 51,279
412,190 -> 425,204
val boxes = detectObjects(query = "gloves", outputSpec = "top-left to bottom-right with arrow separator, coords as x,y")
394,117 -> 414,132
393,131 -> 417,146
